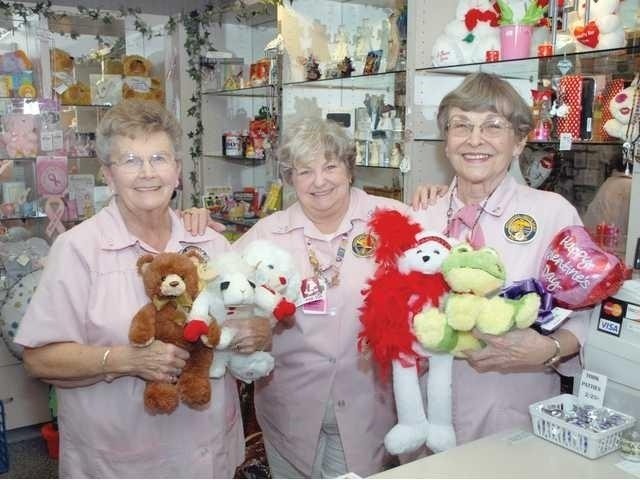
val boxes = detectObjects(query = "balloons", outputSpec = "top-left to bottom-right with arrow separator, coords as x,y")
504,222 -> 624,311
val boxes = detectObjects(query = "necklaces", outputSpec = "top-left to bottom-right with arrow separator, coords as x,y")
446,188 -> 499,243
302,228 -> 351,286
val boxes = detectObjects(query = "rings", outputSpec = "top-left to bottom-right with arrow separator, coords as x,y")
237,343 -> 239,349
168,377 -> 174,384
183,212 -> 192,216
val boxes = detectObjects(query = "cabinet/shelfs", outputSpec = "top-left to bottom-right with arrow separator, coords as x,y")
0,288 -> 54,432
180,0 -> 640,270
1,4 -> 176,222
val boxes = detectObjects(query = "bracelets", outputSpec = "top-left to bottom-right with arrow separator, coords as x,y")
544,337 -> 562,368
102,347 -> 114,383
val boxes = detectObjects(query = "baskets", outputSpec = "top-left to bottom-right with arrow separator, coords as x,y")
527,393 -> 636,460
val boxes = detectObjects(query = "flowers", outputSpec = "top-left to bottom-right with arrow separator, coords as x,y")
464,0 -> 566,33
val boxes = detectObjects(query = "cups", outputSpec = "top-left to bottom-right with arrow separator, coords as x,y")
623,29 -> 640,47
485,49 -> 499,61
537,44 -> 552,56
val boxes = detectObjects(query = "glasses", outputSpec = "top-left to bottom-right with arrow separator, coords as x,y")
445,120 -> 514,138
107,152 -> 178,173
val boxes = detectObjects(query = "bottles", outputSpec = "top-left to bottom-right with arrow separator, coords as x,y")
240,130 -> 250,156
83,195 -> 94,219
368,129 -> 404,168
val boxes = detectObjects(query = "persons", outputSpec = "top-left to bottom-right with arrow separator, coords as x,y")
580,156 -> 633,260
176,113 -> 449,478
12,95 -> 273,479
404,72 -> 593,463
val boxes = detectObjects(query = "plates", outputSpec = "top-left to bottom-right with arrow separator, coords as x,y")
1,269 -> 42,363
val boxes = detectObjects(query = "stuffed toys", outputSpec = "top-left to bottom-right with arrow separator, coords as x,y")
397,234 -> 456,356
568,0 -> 627,58
1,113 -> 37,157
51,47 -> 90,106
604,74 -> 640,142
131,231 -> 300,414
358,209 -> 457,456
413,243 -> 546,359
432,1 -> 504,67
121,54 -> 165,104
